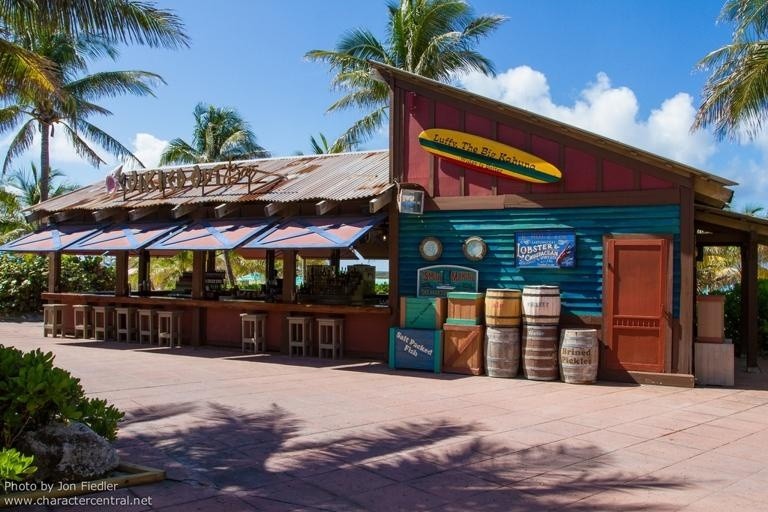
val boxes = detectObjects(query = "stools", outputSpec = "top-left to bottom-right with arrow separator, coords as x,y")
41,302 -> 184,349
239,312 -> 267,354
286,315 -> 345,362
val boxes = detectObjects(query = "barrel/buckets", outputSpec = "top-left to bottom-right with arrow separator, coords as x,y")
522,284 -> 561,326
558,329 -> 600,384
484,288 -> 521,328
522,326 -> 557,380
484,329 -> 520,377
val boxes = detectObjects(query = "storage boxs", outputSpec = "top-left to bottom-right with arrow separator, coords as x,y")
387,291 -> 483,378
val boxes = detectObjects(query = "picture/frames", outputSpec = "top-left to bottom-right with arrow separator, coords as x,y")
512,226 -> 578,271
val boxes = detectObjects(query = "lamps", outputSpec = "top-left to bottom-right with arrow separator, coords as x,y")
396,188 -> 426,215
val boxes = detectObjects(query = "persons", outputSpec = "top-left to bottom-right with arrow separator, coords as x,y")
295,268 -> 303,288
